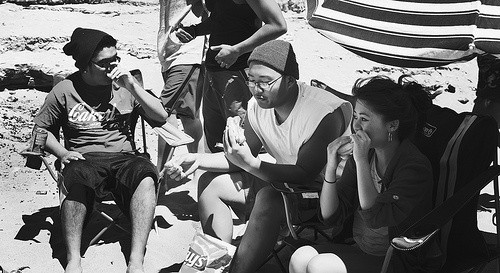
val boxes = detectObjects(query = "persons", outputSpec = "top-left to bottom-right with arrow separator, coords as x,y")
175,0 -> 288,151
156,0 -> 206,155
33,27 -> 168,272
289,73 -> 435,273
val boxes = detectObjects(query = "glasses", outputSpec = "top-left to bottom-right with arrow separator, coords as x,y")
244,73 -> 287,89
89,55 -> 121,69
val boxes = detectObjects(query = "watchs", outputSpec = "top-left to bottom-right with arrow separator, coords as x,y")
165,39 -> 352,272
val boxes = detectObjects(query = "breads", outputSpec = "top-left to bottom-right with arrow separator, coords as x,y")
337,139 -> 354,155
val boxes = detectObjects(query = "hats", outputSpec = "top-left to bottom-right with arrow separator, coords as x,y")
247,39 -> 300,81
62,27 -> 108,70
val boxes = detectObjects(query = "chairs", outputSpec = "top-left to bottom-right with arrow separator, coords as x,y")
241,77 -> 500,273
20,67 -> 196,250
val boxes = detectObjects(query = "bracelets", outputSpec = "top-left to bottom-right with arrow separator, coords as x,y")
323,175 -> 338,184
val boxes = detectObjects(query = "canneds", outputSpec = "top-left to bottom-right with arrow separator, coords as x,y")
30,128 -> 47,153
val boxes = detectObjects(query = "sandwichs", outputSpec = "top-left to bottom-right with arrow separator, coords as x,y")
176,28 -> 193,41
227,116 -> 246,144
